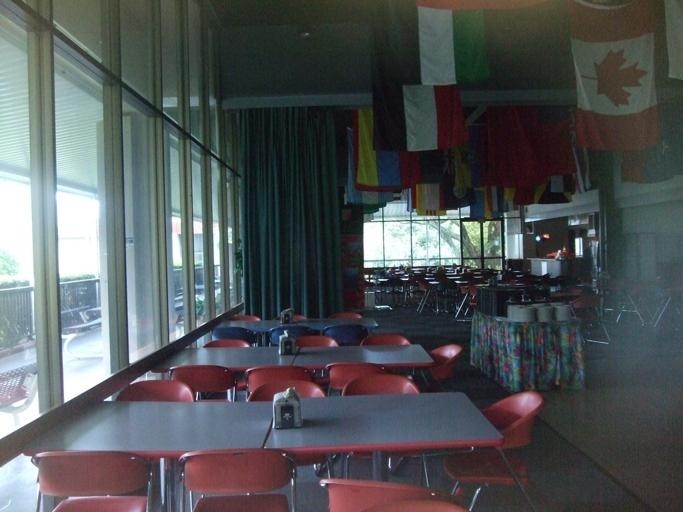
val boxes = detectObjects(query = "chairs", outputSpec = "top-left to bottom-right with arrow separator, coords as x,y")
168,365 -> 238,479
363,262 -> 682,352
339,374 -> 429,485
284,334 -> 339,349
244,365 -> 320,472
243,381 -> 330,500
441,389 -> 544,507
209,310 -> 379,342
323,361 -> 395,464
318,469 -> 468,510
172,449 -> 290,510
203,339 -> 253,350
29,448 -> 156,512
423,343 -> 461,394
113,379 -> 193,510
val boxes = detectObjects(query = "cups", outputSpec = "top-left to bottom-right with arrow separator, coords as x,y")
507,301 -> 571,322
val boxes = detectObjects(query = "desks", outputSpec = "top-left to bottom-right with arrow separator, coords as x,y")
150,342 -> 439,378
25,392 -> 504,471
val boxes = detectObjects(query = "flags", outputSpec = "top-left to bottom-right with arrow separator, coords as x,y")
339,1 -> 682,223
